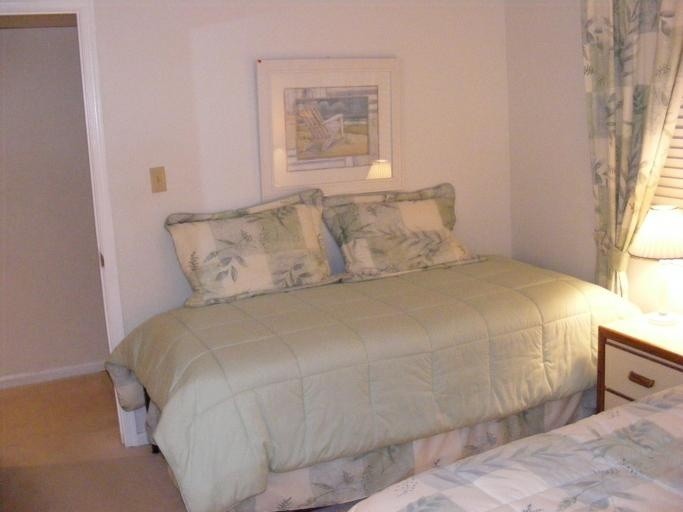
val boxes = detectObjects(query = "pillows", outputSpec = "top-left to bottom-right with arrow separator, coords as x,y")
320,183 -> 489,285
164,188 -> 351,307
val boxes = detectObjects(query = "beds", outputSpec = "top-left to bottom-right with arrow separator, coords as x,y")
125,251 -> 637,512
347,384 -> 683,512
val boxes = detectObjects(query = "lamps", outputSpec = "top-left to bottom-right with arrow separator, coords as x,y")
628,206 -> 683,320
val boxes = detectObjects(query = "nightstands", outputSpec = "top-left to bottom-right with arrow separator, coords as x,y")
592,318 -> 683,414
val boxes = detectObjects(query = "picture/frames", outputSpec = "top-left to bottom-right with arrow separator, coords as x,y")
255,57 -> 405,204
